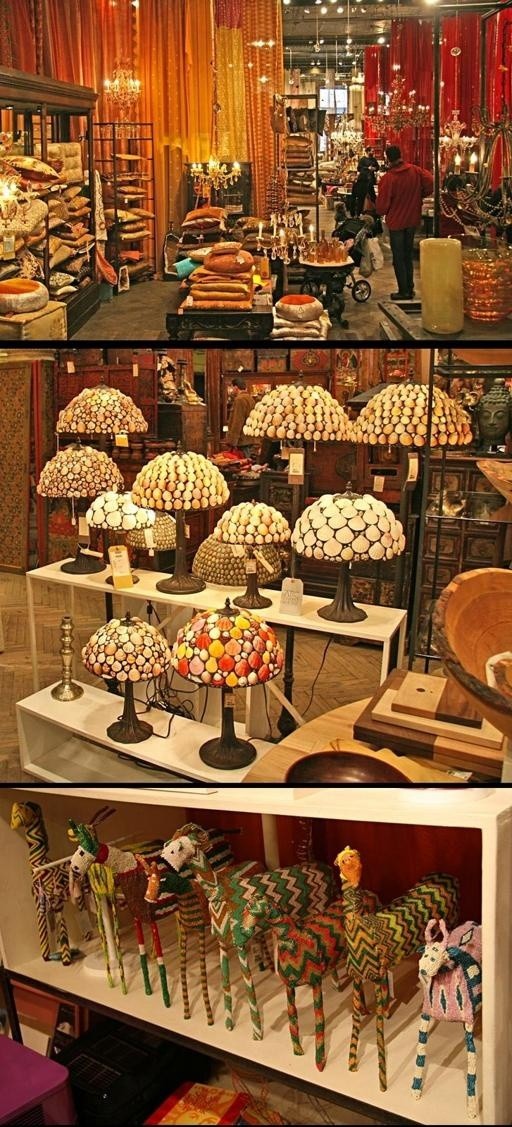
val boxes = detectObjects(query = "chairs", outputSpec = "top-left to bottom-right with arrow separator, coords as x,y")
1,954 -> 76,1124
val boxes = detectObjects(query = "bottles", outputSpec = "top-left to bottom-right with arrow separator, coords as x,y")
300,229 -> 348,264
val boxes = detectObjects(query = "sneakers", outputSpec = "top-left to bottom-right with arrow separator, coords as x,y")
390,292 -> 413,301
411,291 -> 415,298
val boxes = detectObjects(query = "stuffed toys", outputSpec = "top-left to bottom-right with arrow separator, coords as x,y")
10,801 -> 482,1119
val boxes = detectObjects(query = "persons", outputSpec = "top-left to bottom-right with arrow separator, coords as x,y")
332,147 -> 390,239
374,147 -> 434,300
476,378 -> 512,445
225,378 -> 259,458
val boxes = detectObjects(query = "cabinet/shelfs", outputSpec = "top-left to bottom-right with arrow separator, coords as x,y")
0,50 -> 160,341
2,788 -> 512,1126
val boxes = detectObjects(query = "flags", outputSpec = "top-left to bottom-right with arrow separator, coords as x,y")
10,801 -> 482,1119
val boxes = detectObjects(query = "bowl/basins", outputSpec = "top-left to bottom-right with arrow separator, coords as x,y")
283,749 -> 414,783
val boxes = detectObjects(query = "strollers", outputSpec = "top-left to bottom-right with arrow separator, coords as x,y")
297,208 -> 384,318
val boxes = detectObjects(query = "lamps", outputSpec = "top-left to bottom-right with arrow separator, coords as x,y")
15,345 -> 511,782
166,0 -> 511,340
